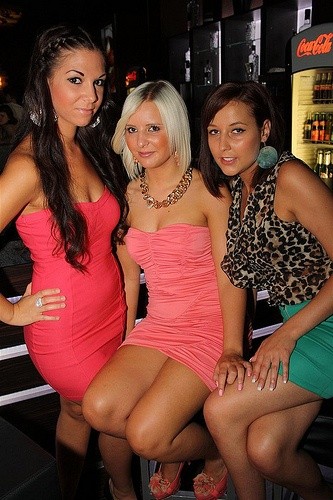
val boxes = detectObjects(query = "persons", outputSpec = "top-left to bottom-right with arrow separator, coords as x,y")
0,23 -> 333,500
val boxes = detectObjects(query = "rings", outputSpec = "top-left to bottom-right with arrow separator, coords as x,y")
35,297 -> 43,307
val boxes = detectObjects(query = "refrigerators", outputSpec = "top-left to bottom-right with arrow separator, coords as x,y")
286,23 -> 333,193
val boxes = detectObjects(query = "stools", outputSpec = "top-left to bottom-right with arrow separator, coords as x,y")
0,414 -> 60,500
135,385 -> 333,500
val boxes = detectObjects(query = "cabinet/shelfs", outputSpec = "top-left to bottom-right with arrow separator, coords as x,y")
224,5 -> 262,92
178,19 -> 221,92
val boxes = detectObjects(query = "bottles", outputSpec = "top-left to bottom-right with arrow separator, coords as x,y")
185,47 -> 191,82
298,9 -> 313,32
303,112 -> 333,144
313,72 -> 333,103
313,147 -> 333,178
204,58 -> 212,86
249,44 -> 259,81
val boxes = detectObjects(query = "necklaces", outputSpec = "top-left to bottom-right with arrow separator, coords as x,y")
139,165 -> 194,209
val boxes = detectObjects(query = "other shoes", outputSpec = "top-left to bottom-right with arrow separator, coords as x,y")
148,459 -> 184,500
108,479 -> 117,500
192,464 -> 229,500
